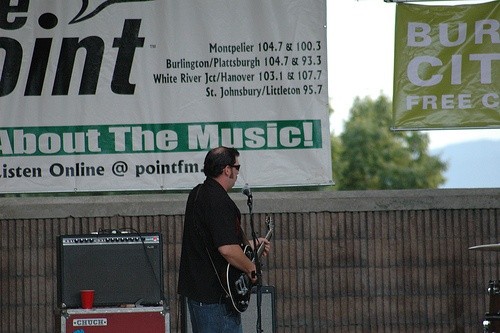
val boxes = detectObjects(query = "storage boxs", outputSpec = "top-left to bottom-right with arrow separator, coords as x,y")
181,286 -> 276,333
55,306 -> 170,333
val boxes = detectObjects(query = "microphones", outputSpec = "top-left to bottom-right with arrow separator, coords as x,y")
241,186 -> 252,199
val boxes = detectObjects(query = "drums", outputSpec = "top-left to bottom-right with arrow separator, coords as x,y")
482,280 -> 500,333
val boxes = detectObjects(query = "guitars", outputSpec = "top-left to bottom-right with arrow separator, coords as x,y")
221,216 -> 273,313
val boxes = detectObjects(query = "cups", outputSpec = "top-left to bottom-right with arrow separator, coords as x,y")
80,289 -> 96,309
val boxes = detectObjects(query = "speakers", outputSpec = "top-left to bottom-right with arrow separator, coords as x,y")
56,232 -> 164,310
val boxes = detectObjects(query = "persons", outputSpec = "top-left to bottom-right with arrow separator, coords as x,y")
174,146 -> 270,333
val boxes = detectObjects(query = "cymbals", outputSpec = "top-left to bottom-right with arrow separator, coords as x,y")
468,243 -> 500,251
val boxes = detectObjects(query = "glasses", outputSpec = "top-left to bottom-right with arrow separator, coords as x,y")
230,164 -> 240,170
